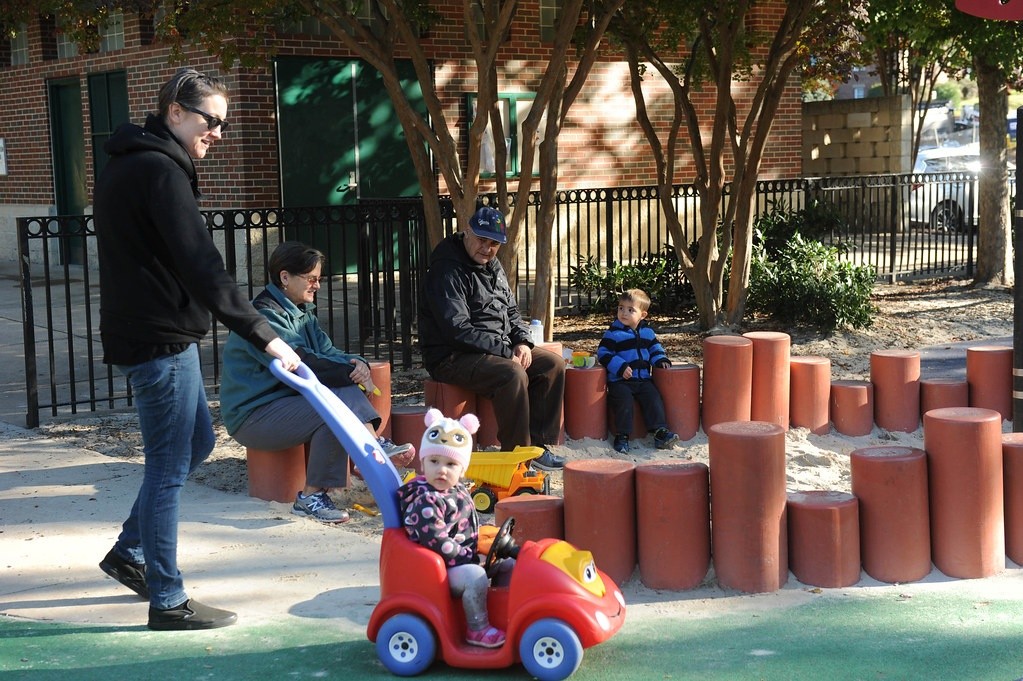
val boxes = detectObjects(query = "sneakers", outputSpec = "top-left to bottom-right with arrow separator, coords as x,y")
292,486 -> 349,522
466,620 -> 507,647
530,444 -> 567,471
613,433 -> 628,454
654,427 -> 679,448
354,437 -> 416,474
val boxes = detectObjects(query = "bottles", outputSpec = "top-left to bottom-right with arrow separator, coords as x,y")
529,319 -> 544,346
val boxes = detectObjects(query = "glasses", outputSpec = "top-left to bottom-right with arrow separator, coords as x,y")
180,102 -> 229,134
289,272 -> 324,285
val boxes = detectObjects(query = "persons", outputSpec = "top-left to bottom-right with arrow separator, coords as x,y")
417,207 -> 566,473
219,242 -> 415,523
99,68 -> 300,631
396,409 -> 517,648
596,288 -> 679,455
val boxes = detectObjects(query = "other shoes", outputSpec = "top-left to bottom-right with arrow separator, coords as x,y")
99,545 -> 154,597
148,594 -> 236,630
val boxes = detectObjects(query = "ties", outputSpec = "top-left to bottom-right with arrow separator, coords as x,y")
469,207 -> 508,245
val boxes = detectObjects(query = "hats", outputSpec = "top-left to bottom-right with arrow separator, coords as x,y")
419,408 -> 480,471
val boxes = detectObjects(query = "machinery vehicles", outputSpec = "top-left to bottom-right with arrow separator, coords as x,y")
463,443 -> 552,513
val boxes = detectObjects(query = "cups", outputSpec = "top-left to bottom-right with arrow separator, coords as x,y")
571,351 -> 590,368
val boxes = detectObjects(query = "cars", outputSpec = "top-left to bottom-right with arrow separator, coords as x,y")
367,516 -> 627,681
906,102 -> 1017,236
